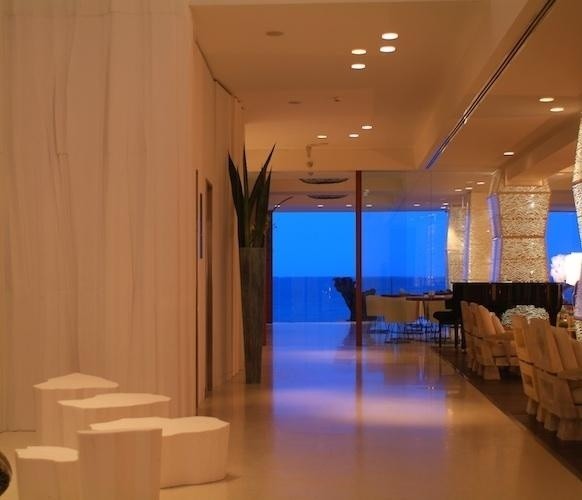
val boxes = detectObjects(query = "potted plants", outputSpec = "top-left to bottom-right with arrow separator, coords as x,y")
226,138 -> 294,386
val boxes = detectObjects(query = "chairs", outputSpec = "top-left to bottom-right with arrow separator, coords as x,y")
363,289 -> 582,443
